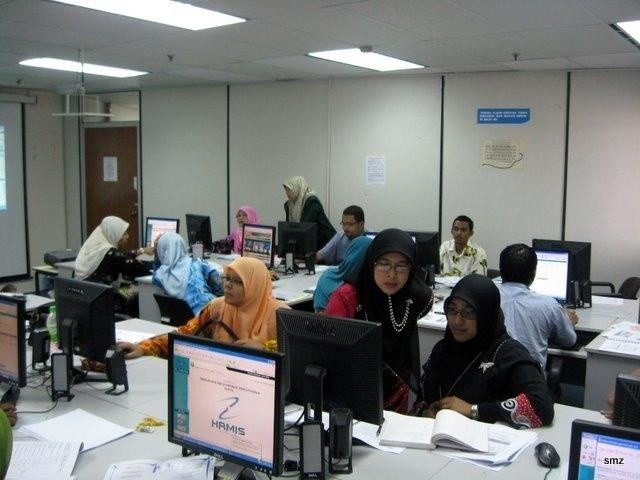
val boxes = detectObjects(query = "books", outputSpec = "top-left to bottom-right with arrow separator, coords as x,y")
378,405 -> 490,453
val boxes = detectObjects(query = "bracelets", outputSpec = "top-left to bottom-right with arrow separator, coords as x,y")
471,402 -> 479,420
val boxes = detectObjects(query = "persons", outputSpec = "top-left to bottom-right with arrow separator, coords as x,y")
0,402 -> 17,426
283,176 -> 337,250
440,215 -> 487,278
314,206 -> 364,264
211,205 -> 260,255
414,274 -> 554,429
0,404 -> 12,480
600,365 -> 640,419
313,237 -> 374,315
152,233 -> 224,317
115,257 -> 292,359
493,243 -> 579,382
72,216 -> 154,313
324,228 -> 434,416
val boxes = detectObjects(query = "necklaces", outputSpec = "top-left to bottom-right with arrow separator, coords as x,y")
386,292 -> 412,333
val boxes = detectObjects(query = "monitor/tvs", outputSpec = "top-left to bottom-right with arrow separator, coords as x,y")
612,373 -> 640,429
276,307 -> 384,445
0,292 -> 27,405
278,221 -> 317,269
362,230 -> 592,306
145,217 -> 180,255
568,419 -> 639,480
241,223 -> 276,270
186,213 -> 213,258
168,332 -> 285,480
54,277 -> 116,381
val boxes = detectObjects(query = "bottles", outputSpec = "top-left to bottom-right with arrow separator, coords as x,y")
46,305 -> 62,356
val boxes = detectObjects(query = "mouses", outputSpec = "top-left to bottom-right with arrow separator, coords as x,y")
535,442 -> 560,468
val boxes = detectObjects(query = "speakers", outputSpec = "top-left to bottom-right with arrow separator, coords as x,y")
330,407 -> 353,474
304,252 -> 316,275
32,328 -> 51,372
300,421 -> 325,480
105,345 -> 128,395
285,251 -> 295,275
51,353 -> 75,401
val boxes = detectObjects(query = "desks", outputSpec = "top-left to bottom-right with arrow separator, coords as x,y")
135,254 -> 640,411
31,261 -> 75,294
0,317 -> 639,480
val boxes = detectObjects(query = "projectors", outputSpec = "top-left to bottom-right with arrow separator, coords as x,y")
16,294 -> 55,327
44,250 -> 80,267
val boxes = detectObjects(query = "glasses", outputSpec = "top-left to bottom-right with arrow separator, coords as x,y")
373,262 -> 412,274
221,276 -> 242,286
340,221 -> 358,226
445,307 -> 475,320
